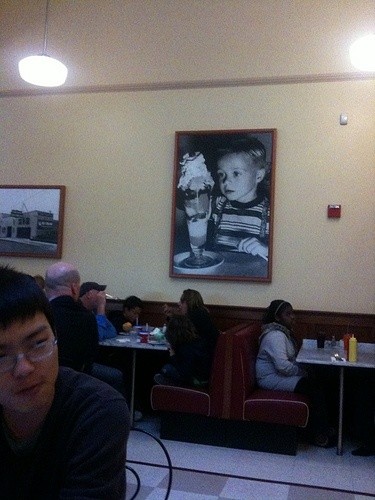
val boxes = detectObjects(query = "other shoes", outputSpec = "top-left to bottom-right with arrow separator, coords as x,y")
350,443 -> 375,457
312,433 -> 337,449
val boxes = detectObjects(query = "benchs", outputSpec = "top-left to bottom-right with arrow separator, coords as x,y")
151,322 -> 309,456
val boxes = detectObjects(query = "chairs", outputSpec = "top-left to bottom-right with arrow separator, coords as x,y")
124,427 -> 172,500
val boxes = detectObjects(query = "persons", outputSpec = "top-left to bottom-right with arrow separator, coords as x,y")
254,299 -> 329,447
153,289 -> 220,387
108,296 -> 142,333
78,282 -> 142,421
351,424 -> 375,456
175,138 -> 270,262
42,262 -> 98,377
0,264 -> 131,500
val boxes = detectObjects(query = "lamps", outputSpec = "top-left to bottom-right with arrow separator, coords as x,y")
18,0 -> 68,88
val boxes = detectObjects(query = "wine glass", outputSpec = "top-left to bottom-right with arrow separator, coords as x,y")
180,187 -> 215,269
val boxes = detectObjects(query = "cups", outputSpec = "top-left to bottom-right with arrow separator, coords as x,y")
139,333 -> 149,343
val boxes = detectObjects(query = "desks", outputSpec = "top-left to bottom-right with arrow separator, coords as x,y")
97,324 -> 170,430
295,340 -> 375,456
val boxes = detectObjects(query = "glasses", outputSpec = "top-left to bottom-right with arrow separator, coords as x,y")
0,337 -> 58,373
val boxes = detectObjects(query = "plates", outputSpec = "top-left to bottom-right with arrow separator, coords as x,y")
173,251 -> 224,271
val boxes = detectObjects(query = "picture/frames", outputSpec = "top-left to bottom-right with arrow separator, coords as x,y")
169,128 -> 277,284
0,184 -> 66,259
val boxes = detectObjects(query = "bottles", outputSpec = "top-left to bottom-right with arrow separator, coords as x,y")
344,334 -> 351,361
349,334 -> 356,363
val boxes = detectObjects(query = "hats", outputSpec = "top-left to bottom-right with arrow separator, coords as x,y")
79,282 -> 107,297
